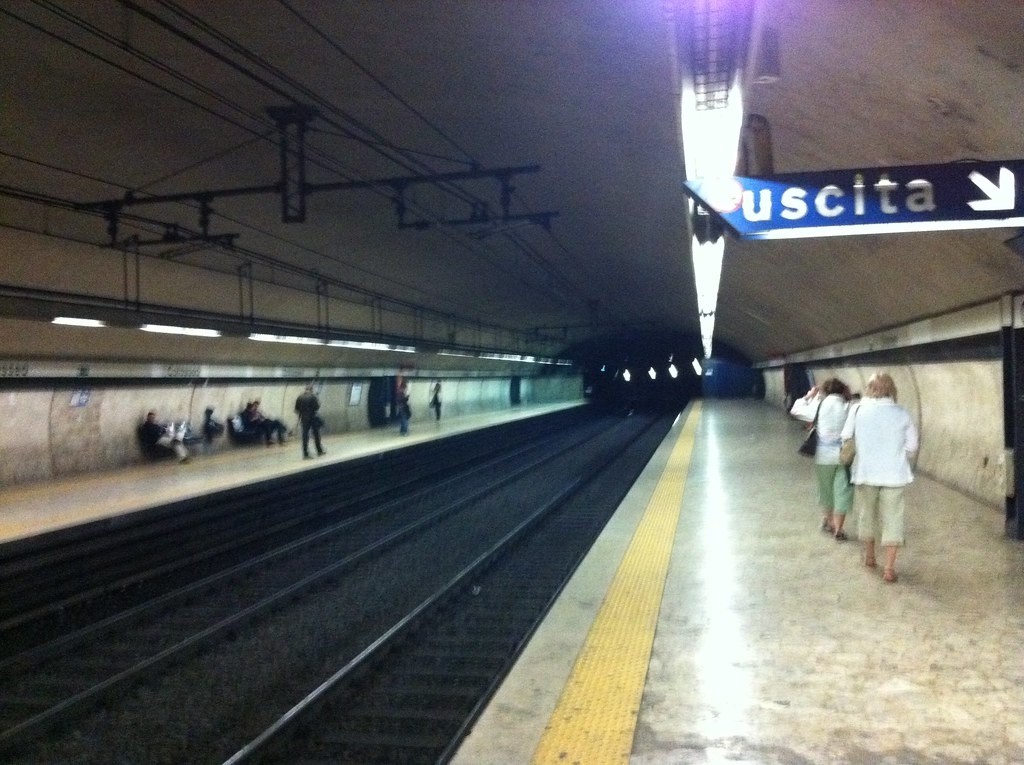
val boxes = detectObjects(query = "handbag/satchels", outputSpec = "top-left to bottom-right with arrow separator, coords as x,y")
165,422 -> 175,440
176,421 -> 186,441
231,415 -> 244,433
313,416 -> 324,431
840,404 -> 860,465
797,399 -> 823,456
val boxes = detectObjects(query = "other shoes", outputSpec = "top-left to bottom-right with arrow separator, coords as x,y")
821,517 -> 834,533
179,456 -> 193,464
883,569 -> 898,582
866,556 -> 877,566
834,531 -> 848,541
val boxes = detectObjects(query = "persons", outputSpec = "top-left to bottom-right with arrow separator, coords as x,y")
202,408 -> 224,442
241,401 -> 286,444
142,411 -> 196,463
840,372 -> 919,581
394,381 -> 412,437
428,383 -> 442,421
294,385 -> 326,459
799,378 -> 860,539
